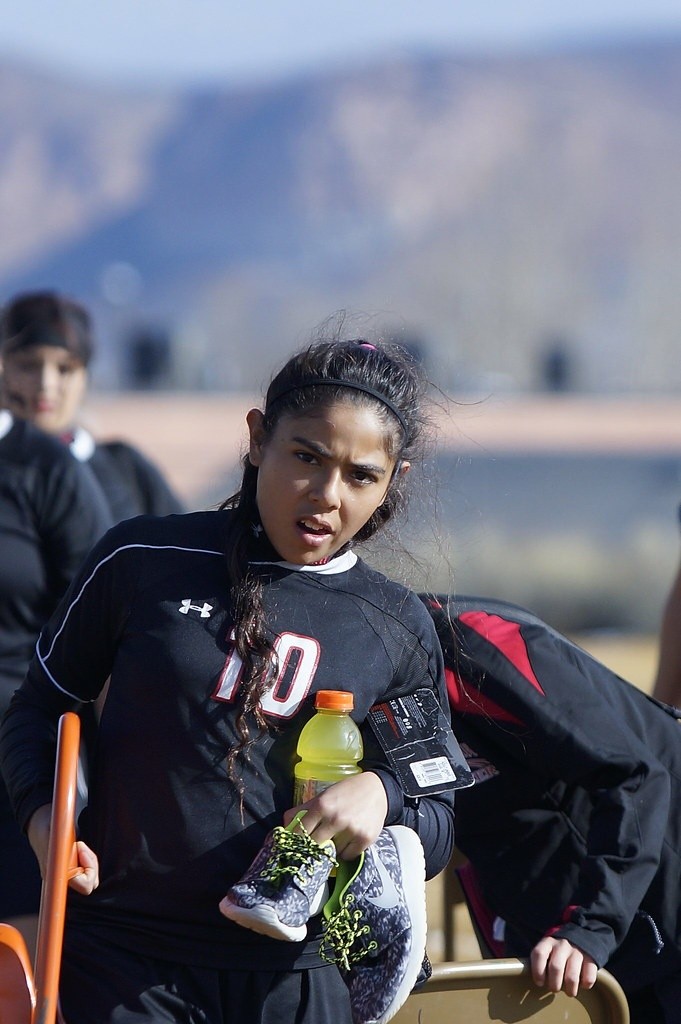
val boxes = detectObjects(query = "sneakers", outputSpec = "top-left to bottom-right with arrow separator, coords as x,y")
318,825 -> 427,1024
218,809 -> 335,943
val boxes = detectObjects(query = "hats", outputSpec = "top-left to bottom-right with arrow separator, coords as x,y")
0,293 -> 94,364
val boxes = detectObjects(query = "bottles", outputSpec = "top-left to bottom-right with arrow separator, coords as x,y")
294,691 -> 364,810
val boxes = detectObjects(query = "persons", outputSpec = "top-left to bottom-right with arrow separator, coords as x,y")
416,590 -> 681,998
1,306 -> 476,1024
0,287 -> 187,523
0,407 -> 116,975
